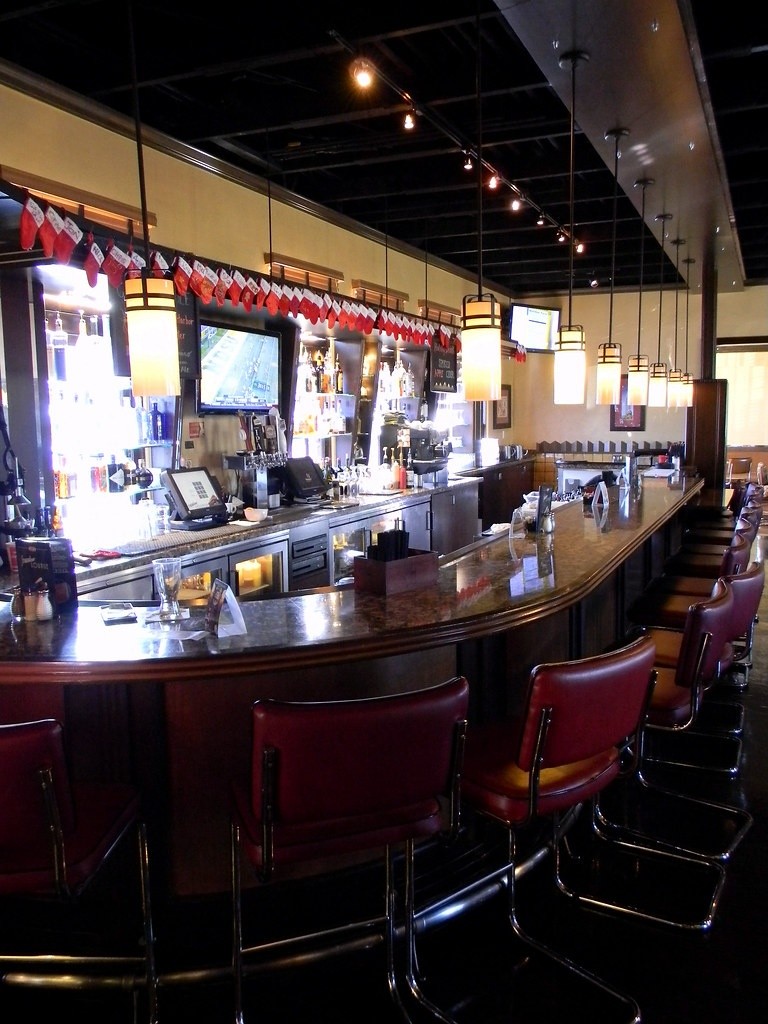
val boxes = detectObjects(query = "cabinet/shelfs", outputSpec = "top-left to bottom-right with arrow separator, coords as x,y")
476,460 -> 536,530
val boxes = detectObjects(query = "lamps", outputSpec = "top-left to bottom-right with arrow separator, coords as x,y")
550,49 -> 590,405
116,0 -> 184,403
590,125 -> 631,412
456,0 -> 503,402
620,175 -> 696,407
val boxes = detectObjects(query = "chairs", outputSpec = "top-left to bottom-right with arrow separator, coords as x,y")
0,716 -> 164,1024
622,457 -> 767,781
226,675 -> 469,1024
403,627 -> 659,1024
549,575 -> 740,933
597,559 -> 767,862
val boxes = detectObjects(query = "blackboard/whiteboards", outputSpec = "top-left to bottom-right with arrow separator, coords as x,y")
175,290 -> 202,380
430,337 -> 458,393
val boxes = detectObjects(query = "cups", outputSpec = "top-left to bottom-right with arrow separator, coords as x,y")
500,444 -> 529,462
6,542 -> 18,574
671,456 -> 679,471
151,558 -> 183,619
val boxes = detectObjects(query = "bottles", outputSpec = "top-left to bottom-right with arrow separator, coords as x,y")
296,343 -> 416,497
45,308 -> 167,499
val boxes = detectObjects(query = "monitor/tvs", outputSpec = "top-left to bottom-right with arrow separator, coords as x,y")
285,457 -> 328,496
160,466 -> 227,520
195,318 -> 283,415
